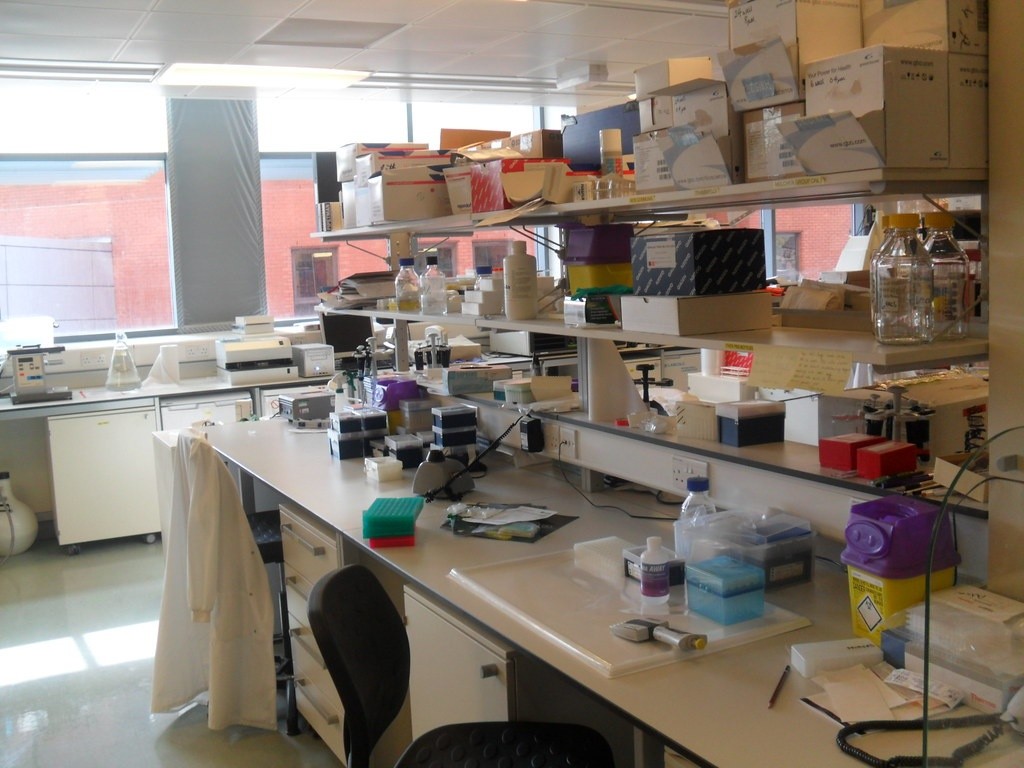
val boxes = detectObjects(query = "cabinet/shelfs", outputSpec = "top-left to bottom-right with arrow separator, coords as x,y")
152,168 -> 1024,768
1,343 -> 702,555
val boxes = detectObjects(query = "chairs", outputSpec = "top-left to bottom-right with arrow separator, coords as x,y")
306,564 -> 614,768
150,430 -> 301,736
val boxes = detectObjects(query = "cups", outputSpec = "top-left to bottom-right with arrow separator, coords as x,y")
700,347 -> 723,376
594,180 -> 635,200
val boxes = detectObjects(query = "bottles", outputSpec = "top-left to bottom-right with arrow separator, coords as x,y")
473,266 -> 503,290
395,258 -> 422,313
641,535 -> 670,607
599,129 -> 623,177
419,256 -> 448,315
501,240 -> 538,321
104,330 -> 143,392
675,476 -> 717,559
869,212 -> 970,345
0,472 -> 38,556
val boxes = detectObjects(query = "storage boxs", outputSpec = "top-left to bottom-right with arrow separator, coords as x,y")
232,314 -> 276,334
290,0 -> 987,498
622,496 -> 1024,716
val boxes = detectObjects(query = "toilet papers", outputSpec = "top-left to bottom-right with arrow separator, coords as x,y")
700,347 -> 726,377
142,344 -> 179,387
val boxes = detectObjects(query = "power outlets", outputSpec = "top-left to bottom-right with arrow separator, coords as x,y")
199,345 -> 211,357
94,353 -> 107,365
183,344 -> 194,358
540,423 -> 559,455
81,352 -> 92,367
671,455 -> 708,493
